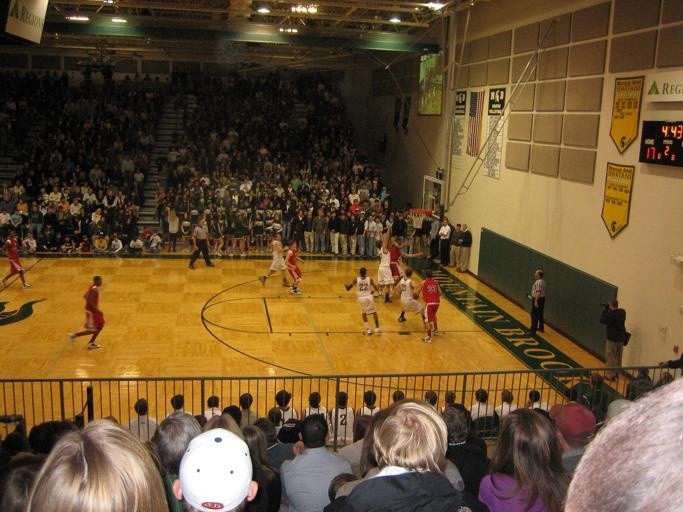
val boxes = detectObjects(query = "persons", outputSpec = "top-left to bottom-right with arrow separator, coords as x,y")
284,240 -> 305,295
259,233 -> 292,287
1,355 -> 683,511
189,219 -> 216,269
371,225 -> 423,303
396,268 -> 426,322
2,229 -> 31,289
600,299 -> 626,381
526,269 -> 547,335
409,208 -> 471,273
415,271 -> 442,343
67,275 -> 105,348
344,267 -> 382,335
1,64 -> 166,257
154,64 -> 417,258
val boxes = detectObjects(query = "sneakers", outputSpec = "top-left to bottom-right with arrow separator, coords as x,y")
23,284 -> 31,288
87,342 -> 101,348
422,337 -> 432,343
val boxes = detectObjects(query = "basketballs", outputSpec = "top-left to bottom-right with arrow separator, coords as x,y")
412,292 -> 418,300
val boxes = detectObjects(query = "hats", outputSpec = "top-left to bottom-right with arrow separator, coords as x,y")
179,427 -> 253,512
549,403 -> 597,443
